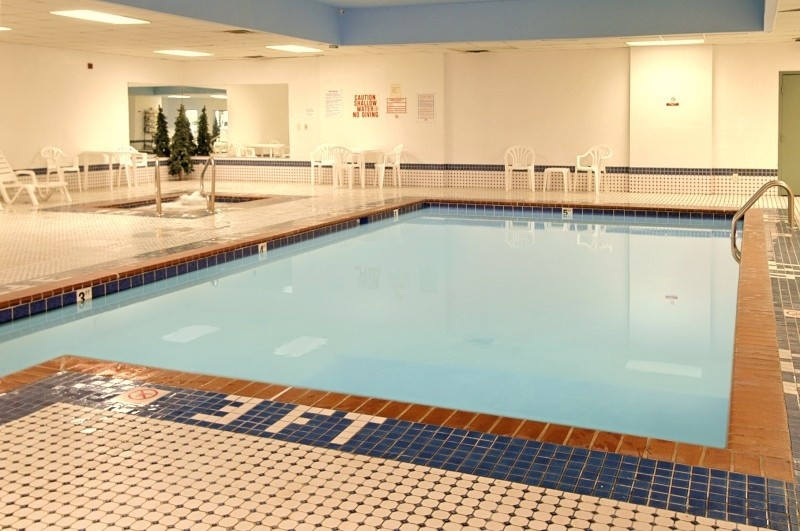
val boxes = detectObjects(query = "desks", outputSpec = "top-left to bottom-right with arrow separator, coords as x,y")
248,144 -> 284,157
80,150 -> 138,192
543,168 -> 570,193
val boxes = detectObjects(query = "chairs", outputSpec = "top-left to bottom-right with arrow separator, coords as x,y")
117,144 -> 150,189
0,147 -> 82,207
504,144 -> 536,192
573,145 -> 613,195
270,141 -> 290,158
311,143 -> 403,190
214,139 -> 257,158
